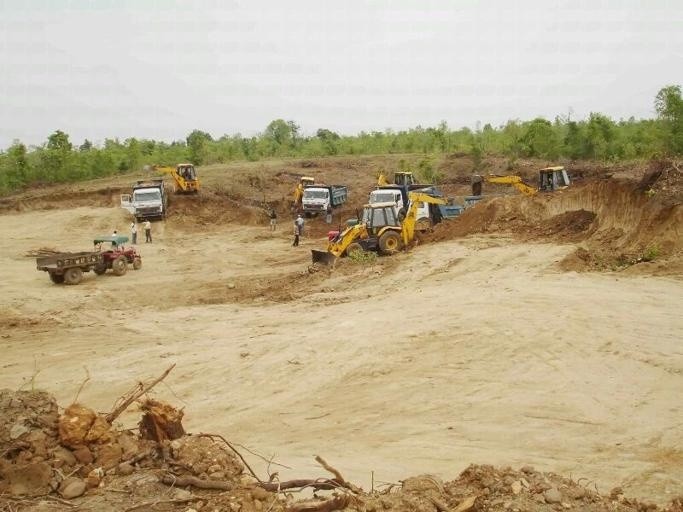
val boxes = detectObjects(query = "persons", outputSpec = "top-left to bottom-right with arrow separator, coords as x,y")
269,209 -> 276,229
295,215 -> 304,235
111,231 -> 119,248
130,221 -> 137,244
183,168 -> 191,180
143,218 -> 152,242
292,221 -> 299,246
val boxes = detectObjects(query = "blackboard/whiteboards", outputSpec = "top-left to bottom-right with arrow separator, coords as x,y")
308,171 -> 491,269
293,177 -> 315,208
142,164 -> 200,194
470,165 -> 573,199
